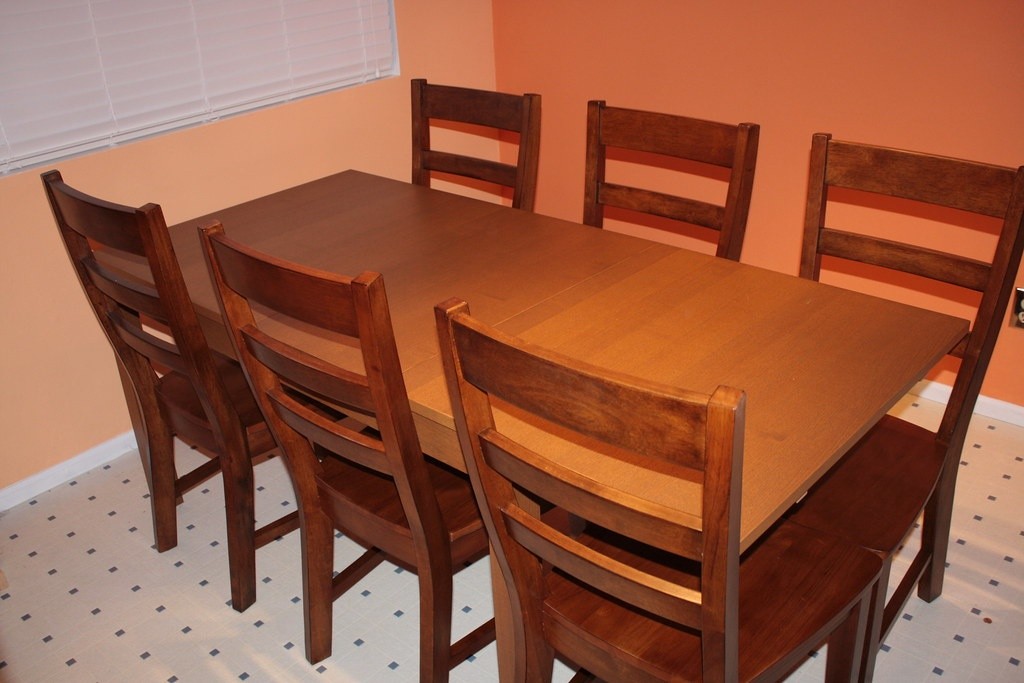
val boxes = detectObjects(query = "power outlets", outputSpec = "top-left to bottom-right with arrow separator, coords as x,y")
1009,287 -> 1024,330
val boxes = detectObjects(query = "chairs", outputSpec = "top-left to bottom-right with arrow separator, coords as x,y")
410,78 -> 543,214
786,132 -> 1024,683
583,100 -> 760,328
197,218 -> 496,683
433,298 -> 884,683
39,170 -> 300,613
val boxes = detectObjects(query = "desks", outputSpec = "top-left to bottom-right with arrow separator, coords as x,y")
93,170 -> 970,556
